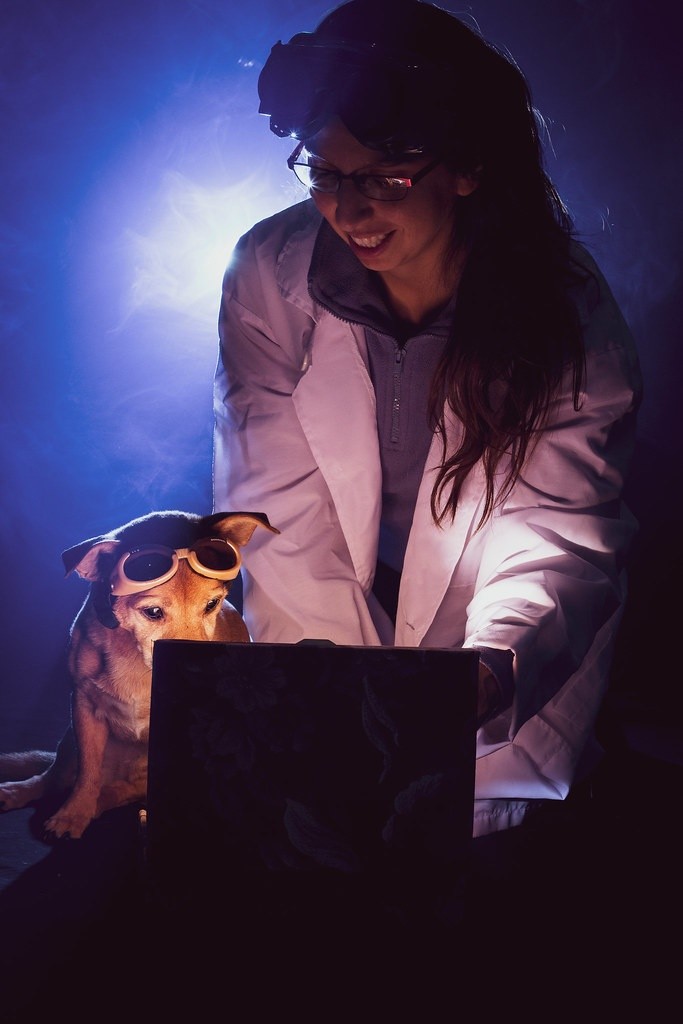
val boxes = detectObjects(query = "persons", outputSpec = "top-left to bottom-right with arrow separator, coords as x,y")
212,0 -> 641,838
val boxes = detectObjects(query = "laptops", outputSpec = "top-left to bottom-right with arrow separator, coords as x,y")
138,639 -> 481,880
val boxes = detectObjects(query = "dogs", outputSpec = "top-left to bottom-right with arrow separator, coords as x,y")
0,511 -> 282,838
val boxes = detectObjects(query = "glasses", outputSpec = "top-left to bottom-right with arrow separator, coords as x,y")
288,141 -> 459,201
108,537 -> 242,597
